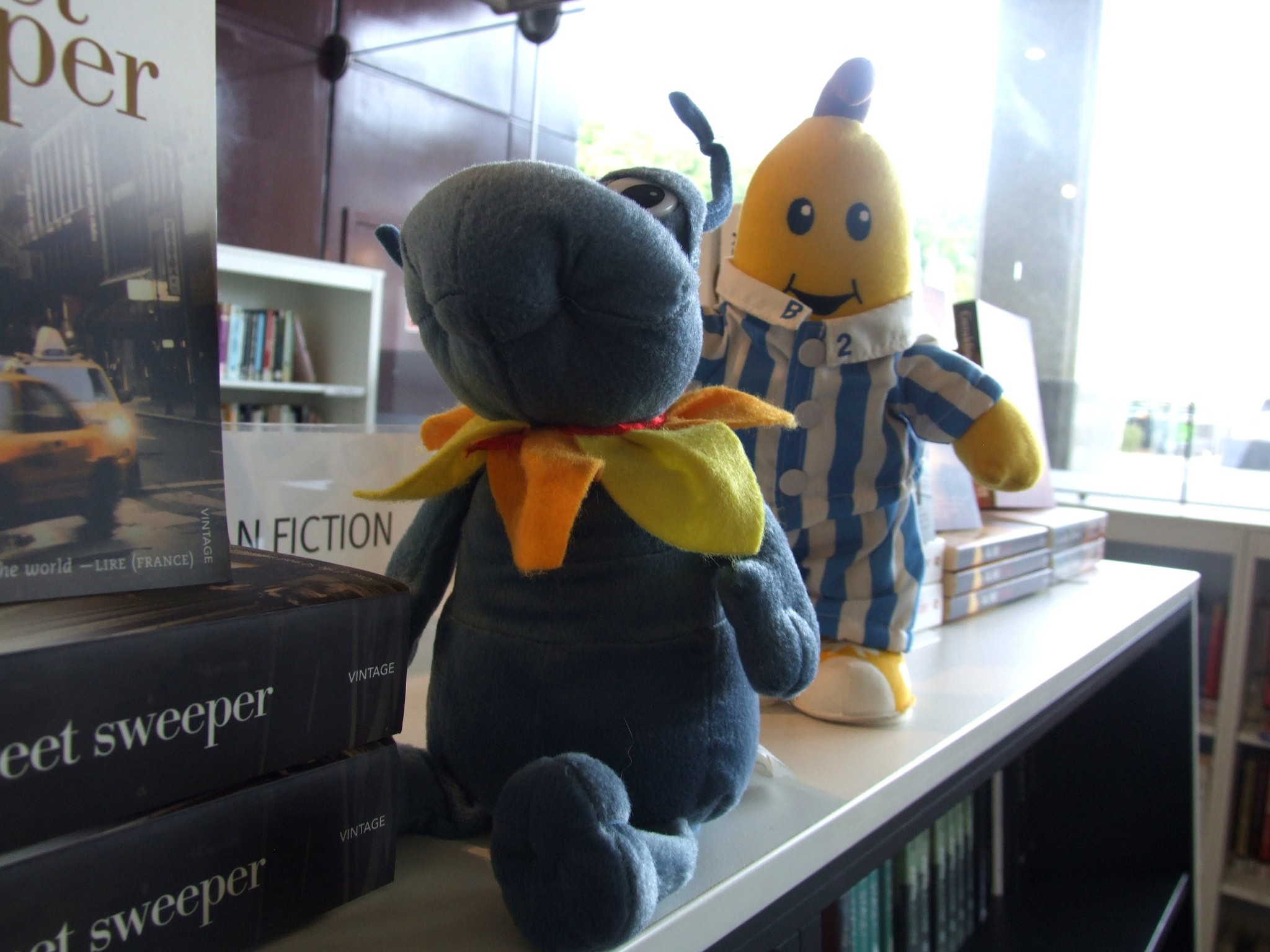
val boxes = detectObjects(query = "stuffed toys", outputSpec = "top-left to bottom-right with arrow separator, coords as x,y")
691,57 -> 1040,724
352,93 -> 820,952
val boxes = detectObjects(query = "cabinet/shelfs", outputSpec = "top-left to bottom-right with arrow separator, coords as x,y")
1054,491 -> 1269,952
265,559 -> 1204,952
215,242 -> 387,432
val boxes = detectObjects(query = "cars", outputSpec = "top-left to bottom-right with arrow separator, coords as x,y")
0,354 -> 122,522
1,324 -> 144,497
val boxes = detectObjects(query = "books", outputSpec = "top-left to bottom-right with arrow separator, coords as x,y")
1201,597 -> 1225,712
838,768 -> 1003,952
221,402 -> 319,431
1246,607 -> 1270,740
219,301 -> 315,381
1198,749 -> 1270,862
915,298 -> 1107,628
